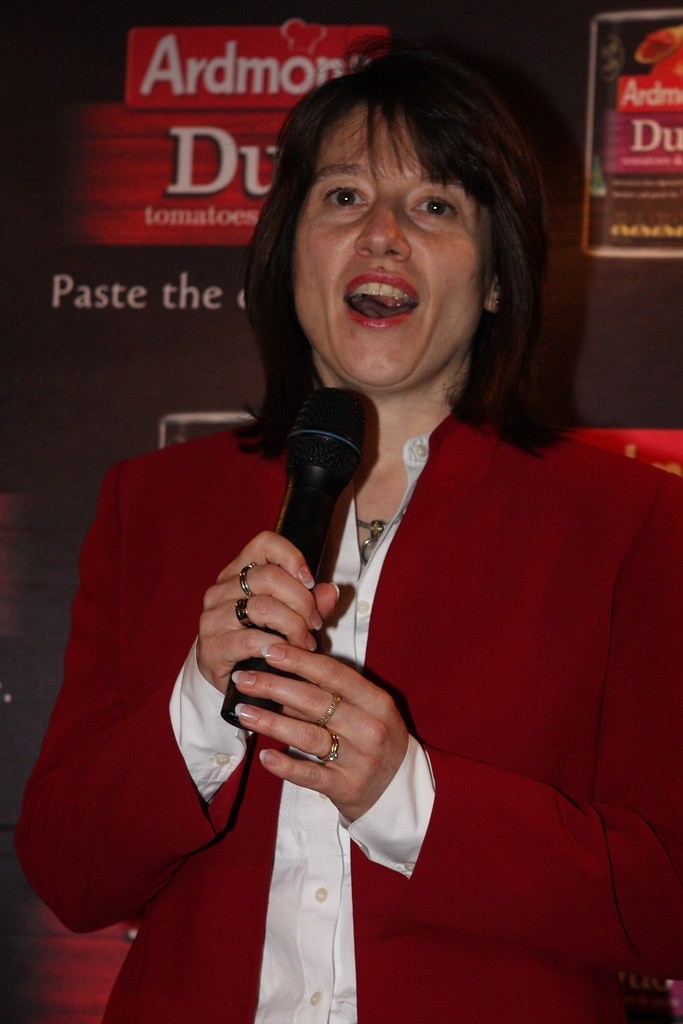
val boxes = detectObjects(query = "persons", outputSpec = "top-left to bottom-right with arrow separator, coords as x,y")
16,29 -> 683,1024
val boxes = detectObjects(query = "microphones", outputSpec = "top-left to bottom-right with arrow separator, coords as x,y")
222,384 -> 365,728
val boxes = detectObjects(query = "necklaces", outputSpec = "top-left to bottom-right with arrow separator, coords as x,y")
355,515 -> 390,567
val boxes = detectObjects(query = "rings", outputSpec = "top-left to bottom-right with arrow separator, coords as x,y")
239,562 -> 257,597
318,729 -> 339,763
316,694 -> 343,726
235,597 -> 257,629
